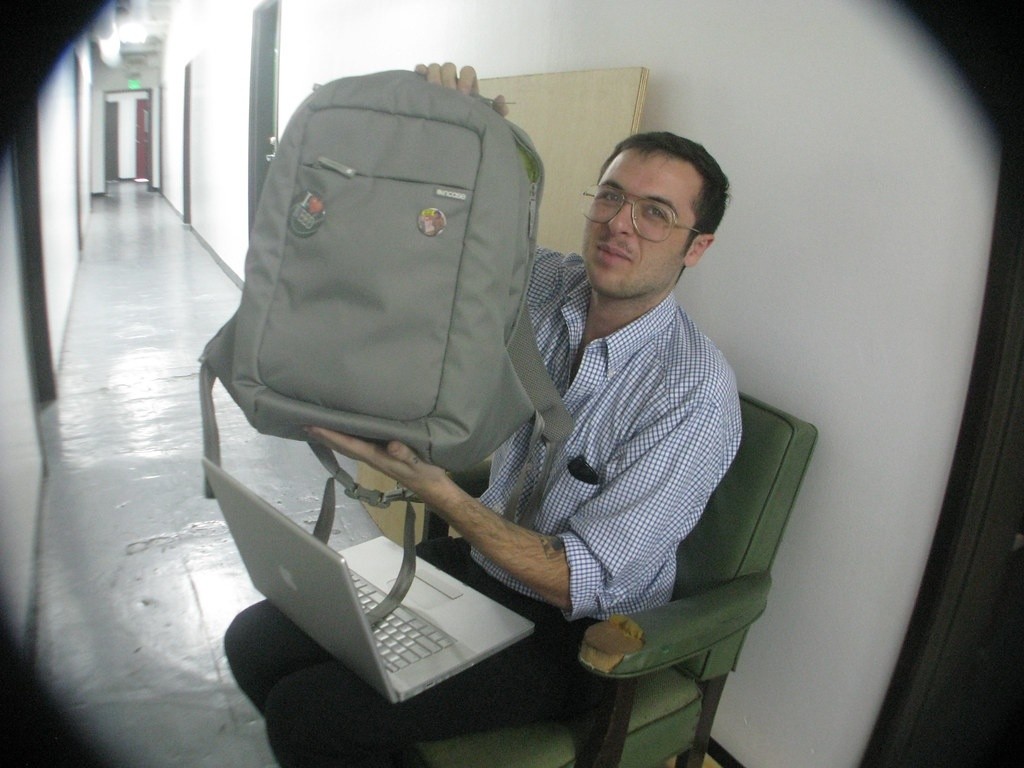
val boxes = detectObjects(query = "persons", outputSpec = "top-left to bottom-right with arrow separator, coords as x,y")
222,59 -> 730,768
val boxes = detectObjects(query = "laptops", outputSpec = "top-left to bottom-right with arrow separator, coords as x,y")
201,455 -> 533,705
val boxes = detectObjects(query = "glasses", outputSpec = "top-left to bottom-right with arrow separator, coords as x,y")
582,185 -> 701,243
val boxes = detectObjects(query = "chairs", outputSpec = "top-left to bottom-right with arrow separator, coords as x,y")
410,393 -> 819,768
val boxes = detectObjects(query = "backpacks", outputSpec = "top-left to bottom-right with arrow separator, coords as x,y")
203,71 -> 544,627
